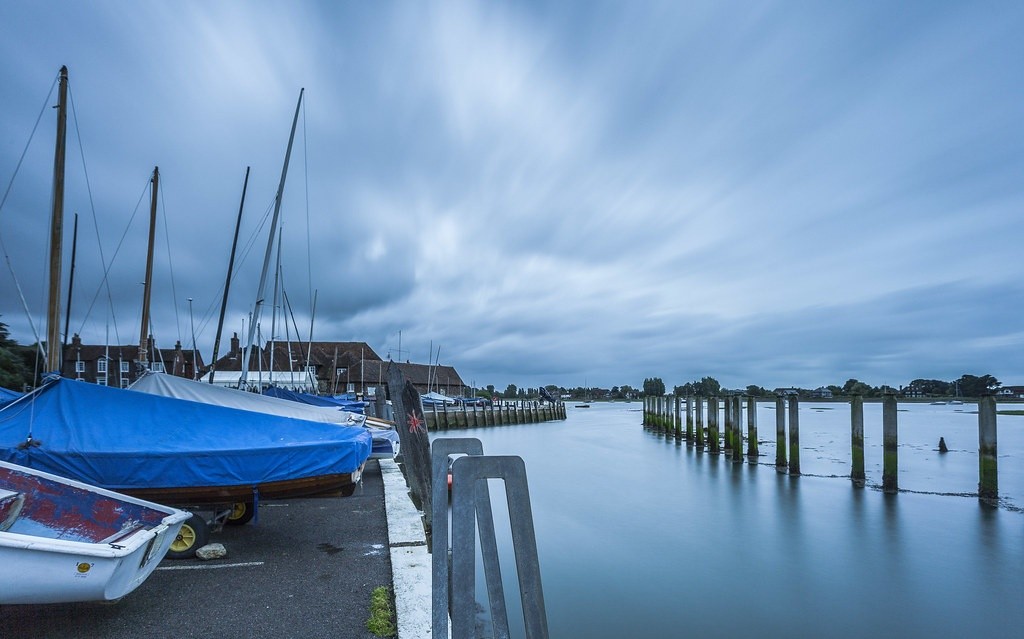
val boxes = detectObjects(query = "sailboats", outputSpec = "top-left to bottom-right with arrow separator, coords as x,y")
574,378 -> 590,408
948,381 -> 964,405
0,64 -> 455,550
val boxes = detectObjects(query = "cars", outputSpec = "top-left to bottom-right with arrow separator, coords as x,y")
465,398 -> 487,407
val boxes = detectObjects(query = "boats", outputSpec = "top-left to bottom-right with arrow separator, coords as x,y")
628,408 -> 643,412
0,460 -> 193,608
929,402 -> 947,405
